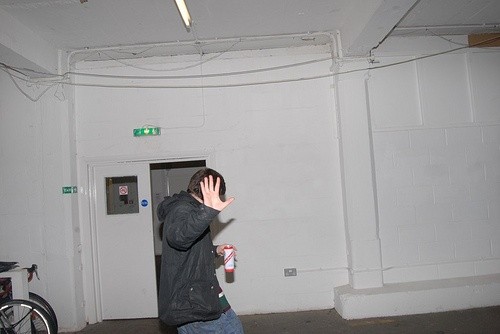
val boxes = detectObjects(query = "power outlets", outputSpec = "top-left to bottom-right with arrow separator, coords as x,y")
284,268 -> 297,276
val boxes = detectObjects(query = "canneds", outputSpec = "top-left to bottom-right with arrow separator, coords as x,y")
223,245 -> 235,272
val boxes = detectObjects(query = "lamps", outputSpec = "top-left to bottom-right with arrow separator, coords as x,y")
175,0 -> 191,29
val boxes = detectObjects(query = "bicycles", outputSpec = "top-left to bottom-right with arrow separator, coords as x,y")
0,259 -> 58,334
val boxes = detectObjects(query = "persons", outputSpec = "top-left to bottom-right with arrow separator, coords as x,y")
157,168 -> 243,334
